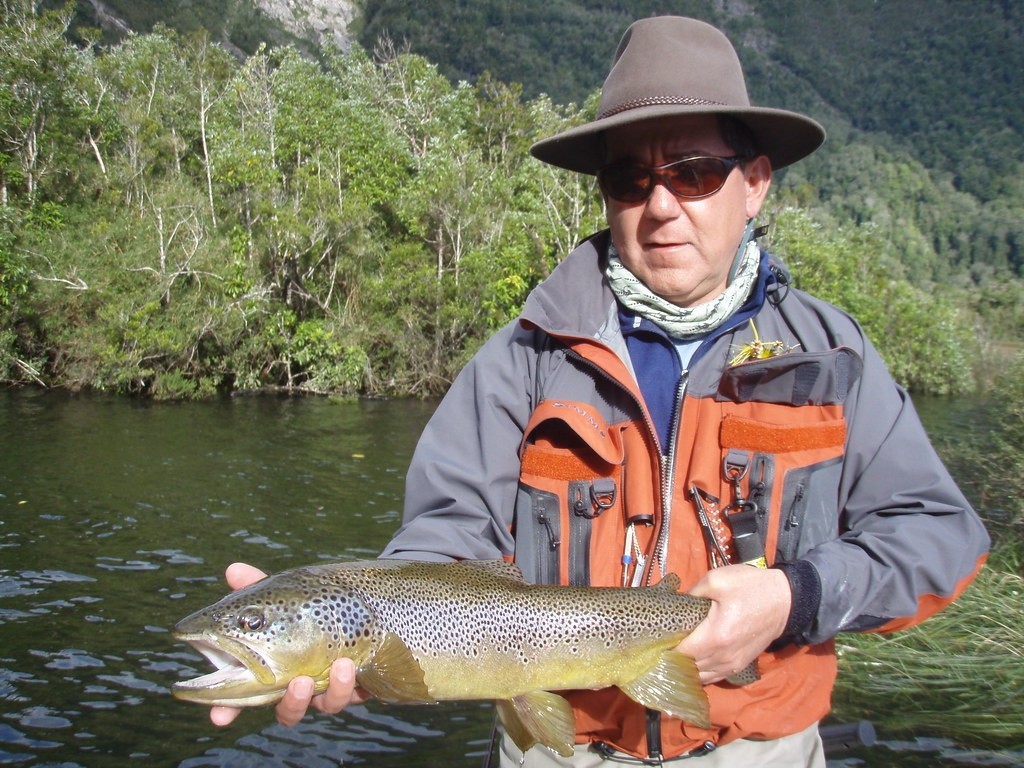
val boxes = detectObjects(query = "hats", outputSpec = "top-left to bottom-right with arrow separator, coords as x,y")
528,13 -> 827,181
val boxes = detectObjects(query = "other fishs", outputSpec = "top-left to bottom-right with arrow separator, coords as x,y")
168,555 -> 768,758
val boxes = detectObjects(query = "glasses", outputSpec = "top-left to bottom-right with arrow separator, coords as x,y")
597,150 -> 753,205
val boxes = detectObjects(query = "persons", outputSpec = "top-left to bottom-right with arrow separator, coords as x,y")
210,15 -> 991,768
